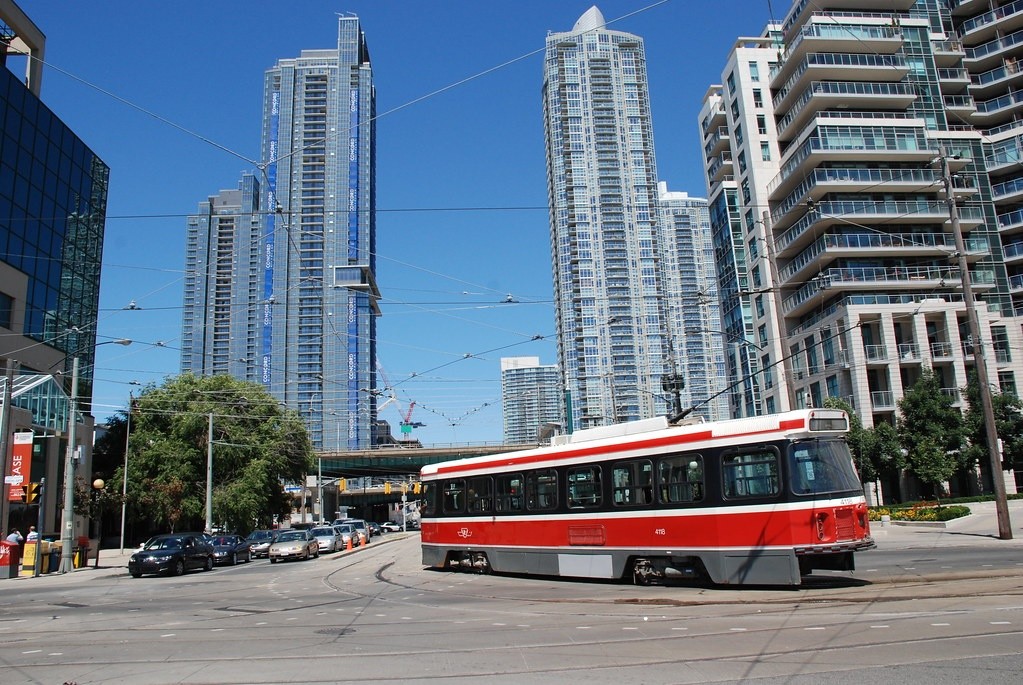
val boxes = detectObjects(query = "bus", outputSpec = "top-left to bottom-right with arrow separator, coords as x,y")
418,405 -> 878,587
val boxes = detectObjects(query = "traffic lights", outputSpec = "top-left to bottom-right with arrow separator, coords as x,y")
28,482 -> 43,505
20,483 -> 32,505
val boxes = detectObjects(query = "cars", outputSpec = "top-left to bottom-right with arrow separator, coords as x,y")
310,526 -> 344,553
268,530 -> 320,563
206,534 -> 252,565
246,521 -> 330,559
333,524 -> 360,547
332,518 -> 419,544
129,536 -> 168,560
128,536 -> 214,578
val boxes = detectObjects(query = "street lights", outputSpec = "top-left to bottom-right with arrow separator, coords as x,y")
93,479 -> 105,569
47,339 -> 135,575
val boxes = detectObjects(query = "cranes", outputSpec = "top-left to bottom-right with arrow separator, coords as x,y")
375,359 -> 418,443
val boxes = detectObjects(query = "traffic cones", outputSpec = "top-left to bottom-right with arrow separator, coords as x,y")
360,535 -> 367,547
346,539 -> 354,551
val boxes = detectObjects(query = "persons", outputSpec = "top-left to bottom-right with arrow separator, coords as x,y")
6,528 -> 23,544
26,526 -> 38,541
